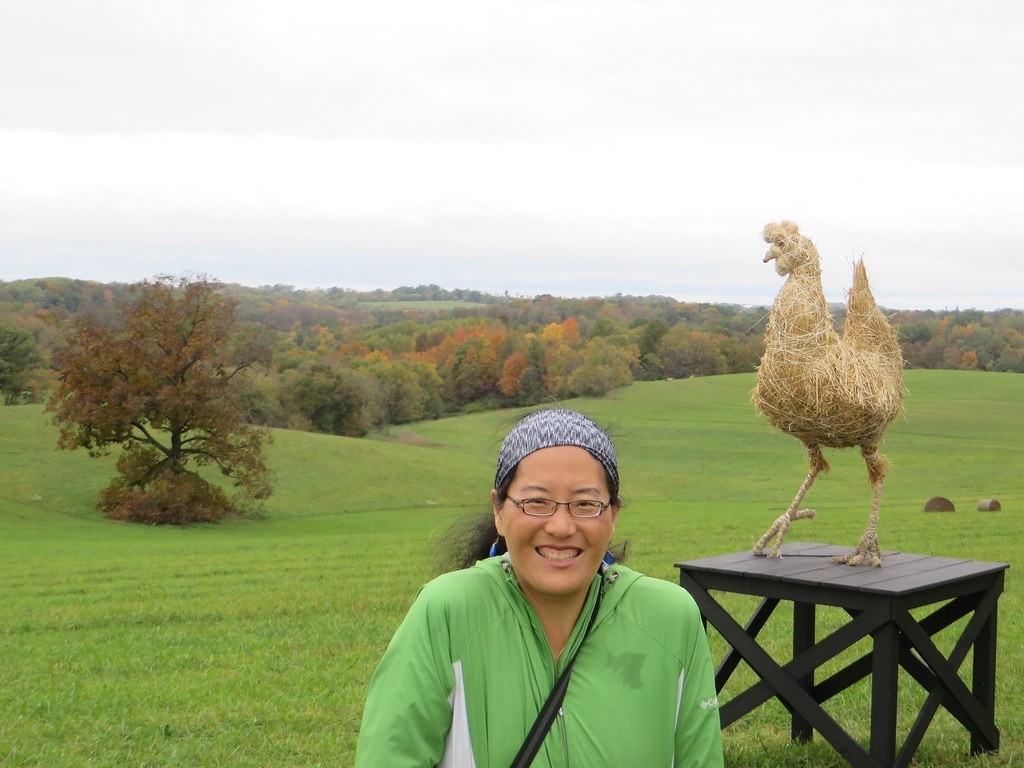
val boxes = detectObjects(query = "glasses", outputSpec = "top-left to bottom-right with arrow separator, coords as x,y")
505,495 -> 611,518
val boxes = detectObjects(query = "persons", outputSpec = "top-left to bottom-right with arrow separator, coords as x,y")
354,407 -> 725,768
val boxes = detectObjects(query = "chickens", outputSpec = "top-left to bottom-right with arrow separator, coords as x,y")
746,220 -> 911,568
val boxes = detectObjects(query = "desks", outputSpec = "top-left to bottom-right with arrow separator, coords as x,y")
673,541 -> 1011,768
